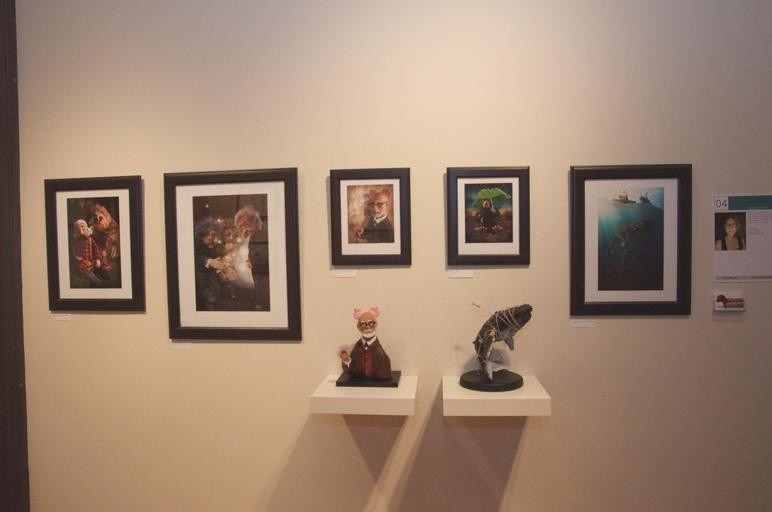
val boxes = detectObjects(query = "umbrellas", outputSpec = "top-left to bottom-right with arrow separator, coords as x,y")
471,188 -> 511,208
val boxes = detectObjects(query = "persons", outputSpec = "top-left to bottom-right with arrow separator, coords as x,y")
337,306 -> 391,380
715,213 -> 746,250
349,191 -> 393,244
191,206 -> 271,312
70,218 -> 108,285
